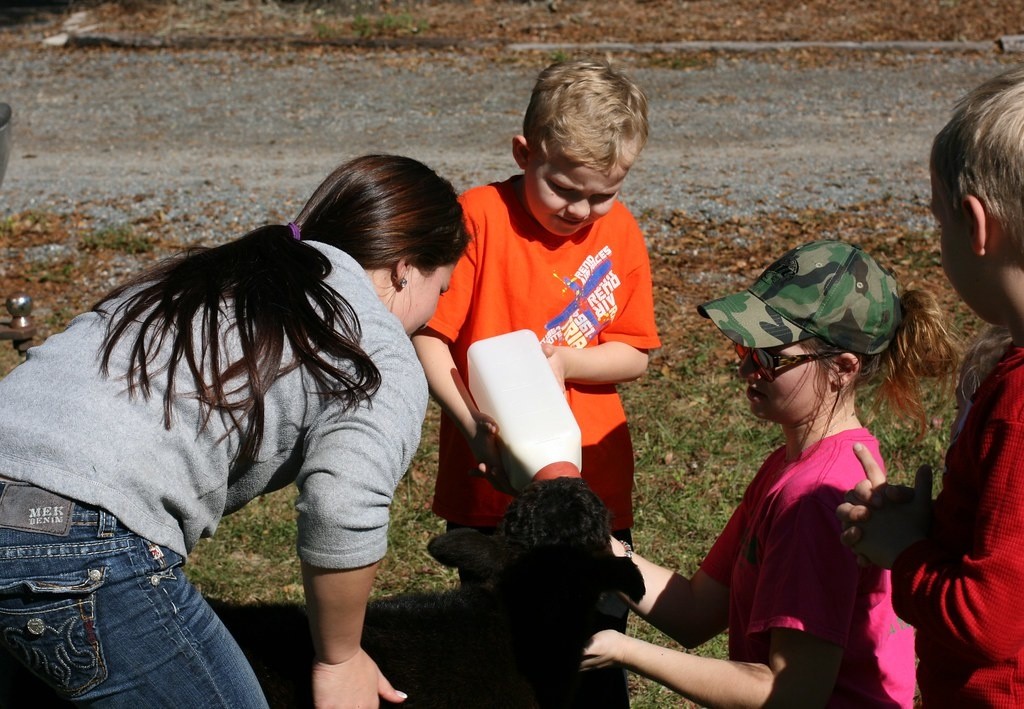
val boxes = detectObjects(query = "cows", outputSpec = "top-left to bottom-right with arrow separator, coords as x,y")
200,475 -> 647,709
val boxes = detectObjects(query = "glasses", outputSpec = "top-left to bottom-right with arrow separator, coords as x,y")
731,341 -> 856,382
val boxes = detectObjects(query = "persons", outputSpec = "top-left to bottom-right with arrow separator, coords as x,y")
411,59 -> 663,709
0,153 -> 473,709
951,328 -> 1013,445
580,241 -> 972,708
835,67 -> 1024,709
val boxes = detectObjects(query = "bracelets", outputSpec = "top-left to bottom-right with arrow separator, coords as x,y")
619,540 -> 632,558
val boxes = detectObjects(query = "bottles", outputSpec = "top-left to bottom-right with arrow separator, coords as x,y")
465,327 -> 583,491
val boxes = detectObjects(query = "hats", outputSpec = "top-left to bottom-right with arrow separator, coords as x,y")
697,239 -> 903,356
